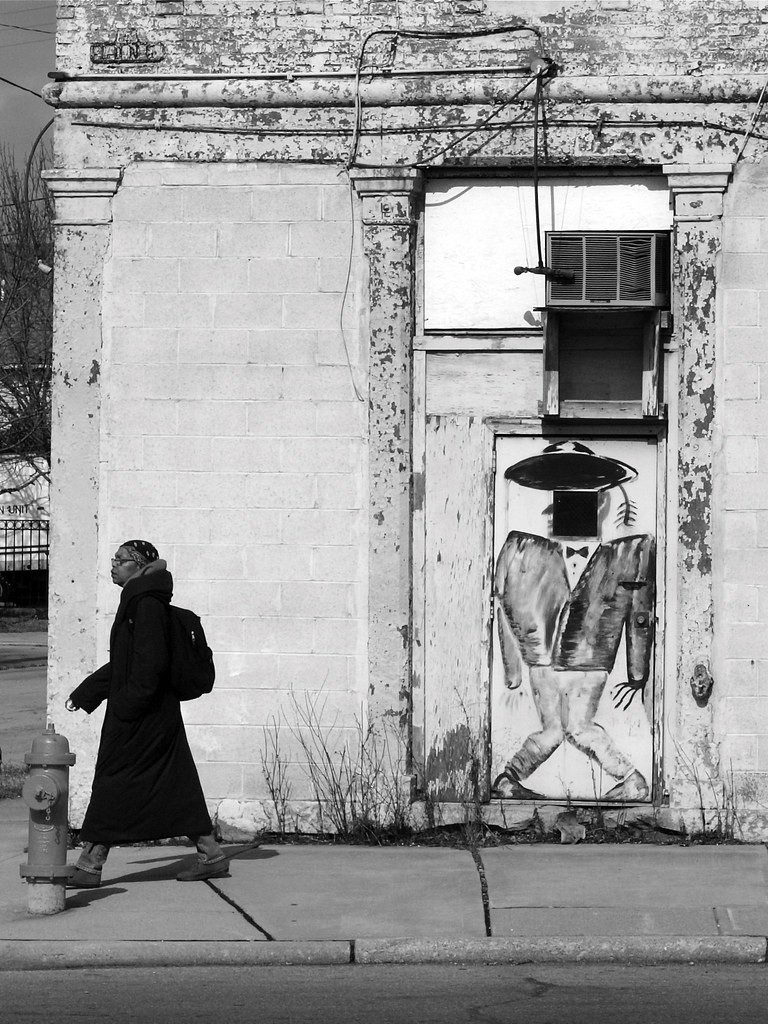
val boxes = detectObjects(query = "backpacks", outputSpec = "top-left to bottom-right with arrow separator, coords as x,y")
127,592 -> 215,700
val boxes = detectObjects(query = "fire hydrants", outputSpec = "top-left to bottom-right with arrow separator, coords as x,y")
21,722 -> 80,916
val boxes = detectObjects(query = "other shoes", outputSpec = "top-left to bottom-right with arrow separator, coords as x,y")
176,857 -> 229,880
64,865 -> 101,888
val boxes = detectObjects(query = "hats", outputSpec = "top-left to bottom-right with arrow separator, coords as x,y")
120,540 -> 159,562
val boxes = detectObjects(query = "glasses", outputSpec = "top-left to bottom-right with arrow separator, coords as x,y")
111,558 -> 134,566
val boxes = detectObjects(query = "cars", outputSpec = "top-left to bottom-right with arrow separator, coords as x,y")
0,453 -> 50,594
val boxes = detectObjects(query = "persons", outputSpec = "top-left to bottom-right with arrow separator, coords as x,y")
65,540 -> 230,886
